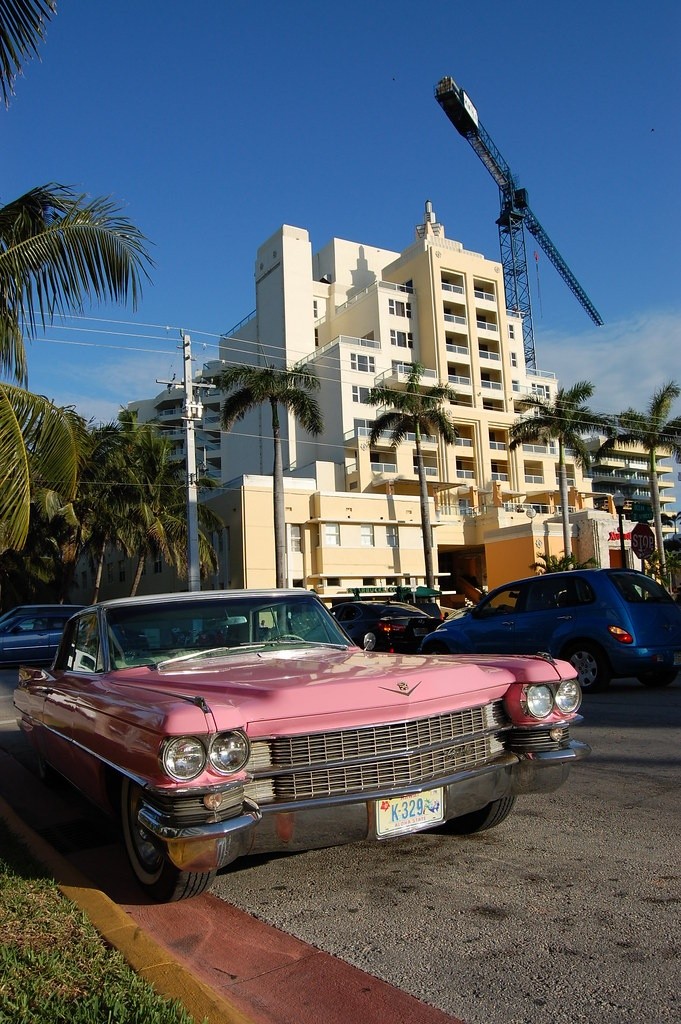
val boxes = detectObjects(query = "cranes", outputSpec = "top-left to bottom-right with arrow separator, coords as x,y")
431,74 -> 603,375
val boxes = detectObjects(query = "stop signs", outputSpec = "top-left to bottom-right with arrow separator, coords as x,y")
631,522 -> 656,560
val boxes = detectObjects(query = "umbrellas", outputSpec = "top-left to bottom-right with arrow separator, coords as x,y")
405,587 -> 441,599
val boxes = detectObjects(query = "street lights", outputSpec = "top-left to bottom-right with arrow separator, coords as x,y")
612,490 -> 629,571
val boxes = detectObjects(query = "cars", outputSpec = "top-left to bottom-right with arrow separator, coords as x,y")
0,604 -> 129,672
298,599 -> 478,655
423,568 -> 681,695
11,587 -> 592,905
